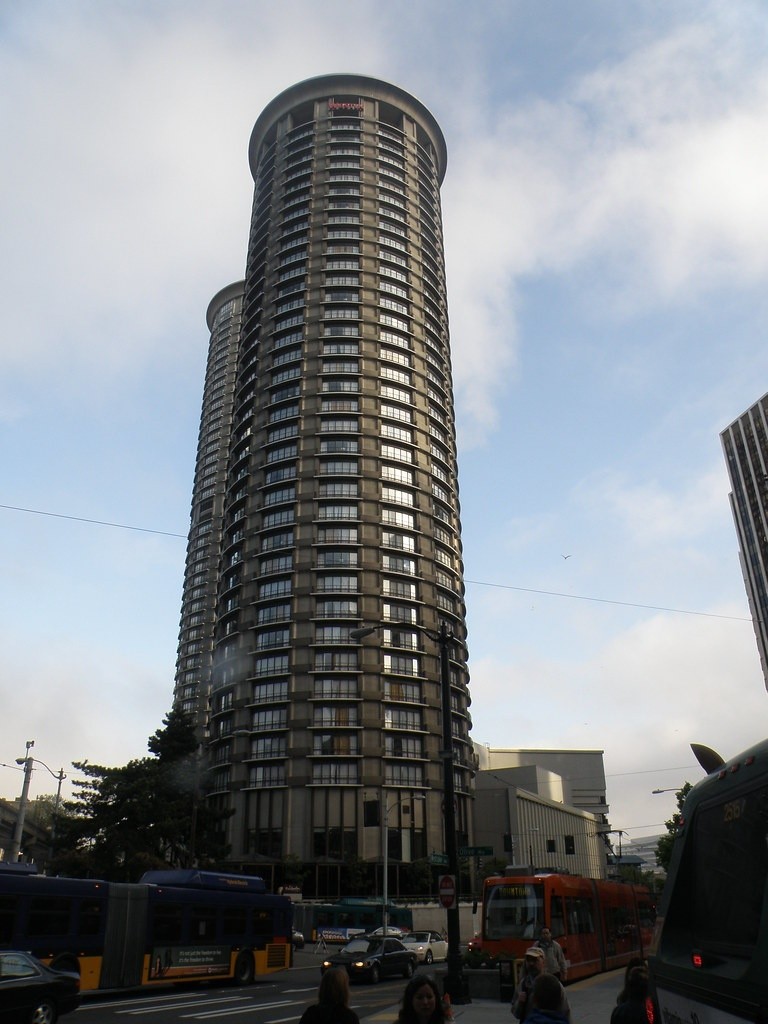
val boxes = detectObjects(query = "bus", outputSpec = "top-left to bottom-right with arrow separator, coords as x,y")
1,861 -> 296,997
472,870 -> 657,986
645,738 -> 768,1024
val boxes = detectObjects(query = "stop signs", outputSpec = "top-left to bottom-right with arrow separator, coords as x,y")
435,874 -> 458,909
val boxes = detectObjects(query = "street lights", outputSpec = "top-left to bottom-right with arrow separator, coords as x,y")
380,795 -> 425,935
17,756 -> 67,870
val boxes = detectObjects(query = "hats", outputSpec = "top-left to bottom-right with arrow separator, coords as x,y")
525,946 -> 544,957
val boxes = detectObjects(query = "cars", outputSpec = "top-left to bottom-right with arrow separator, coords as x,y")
0,949 -> 85,1024
399,930 -> 448,965
321,934 -> 419,985
293,928 -> 305,951
349,925 -> 411,942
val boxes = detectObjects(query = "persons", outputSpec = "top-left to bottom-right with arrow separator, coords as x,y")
297,967 -> 360,1024
610,957 -> 653,1024
521,973 -> 569,1024
511,947 -> 574,1024
532,926 -> 568,982
393,975 -> 444,1024
